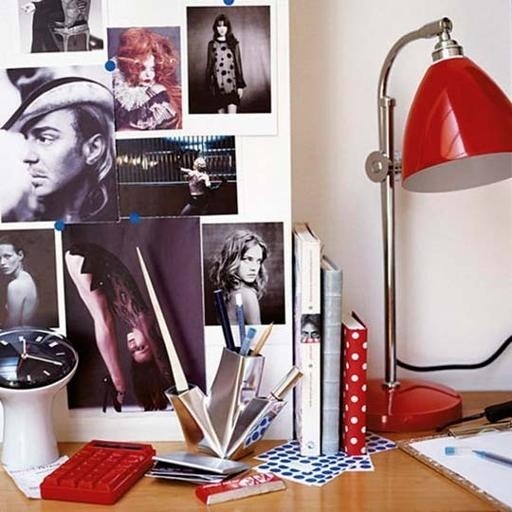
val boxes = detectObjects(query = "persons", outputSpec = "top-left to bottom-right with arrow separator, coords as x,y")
299,313 -> 320,344
0,240 -> 41,329
210,230 -> 267,325
176,157 -> 225,216
111,28 -> 181,130
20,0 -> 65,52
65,243 -> 166,413
205,15 -> 246,113
0,76 -> 115,223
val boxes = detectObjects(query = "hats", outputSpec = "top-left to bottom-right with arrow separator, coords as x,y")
193,157 -> 207,169
0,76 -> 115,133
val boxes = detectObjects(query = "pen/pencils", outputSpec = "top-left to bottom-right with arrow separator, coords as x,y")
444,446 -> 512,469
213,289 -> 277,355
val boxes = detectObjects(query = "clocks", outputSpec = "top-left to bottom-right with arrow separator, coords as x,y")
0,329 -> 81,471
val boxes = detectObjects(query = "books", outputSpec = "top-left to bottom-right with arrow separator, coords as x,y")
294,224 -> 321,459
320,253 -> 343,457
342,308 -> 369,458
396,435 -> 512,512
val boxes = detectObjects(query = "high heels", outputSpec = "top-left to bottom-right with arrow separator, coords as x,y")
102,375 -> 125,412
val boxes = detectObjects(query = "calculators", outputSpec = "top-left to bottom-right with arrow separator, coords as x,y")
40,440 -> 156,504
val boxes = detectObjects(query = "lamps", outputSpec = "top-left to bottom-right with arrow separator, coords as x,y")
367,16 -> 511,433
